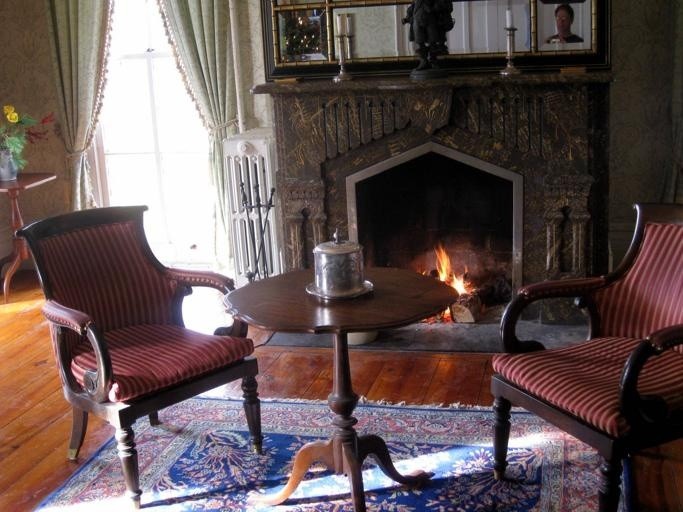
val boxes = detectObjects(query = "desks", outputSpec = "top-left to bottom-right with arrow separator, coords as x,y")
222,265 -> 460,512
0,172 -> 59,306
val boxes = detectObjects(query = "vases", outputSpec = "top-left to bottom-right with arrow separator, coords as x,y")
1,150 -> 19,182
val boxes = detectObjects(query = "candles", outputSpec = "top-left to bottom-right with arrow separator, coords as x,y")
506,10 -> 513,27
345,13 -> 352,35
336,14 -> 343,35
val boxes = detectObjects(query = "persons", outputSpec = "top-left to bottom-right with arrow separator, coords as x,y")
541,3 -> 582,45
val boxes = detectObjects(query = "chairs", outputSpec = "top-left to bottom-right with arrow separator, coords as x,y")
485,201 -> 682,509
13,206 -> 266,512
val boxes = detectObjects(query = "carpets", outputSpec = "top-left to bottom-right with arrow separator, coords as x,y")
32,391 -> 608,511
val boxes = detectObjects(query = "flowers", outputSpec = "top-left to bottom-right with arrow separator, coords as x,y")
1,103 -> 57,167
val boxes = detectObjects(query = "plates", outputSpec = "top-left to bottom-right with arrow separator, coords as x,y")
305,279 -> 374,301
